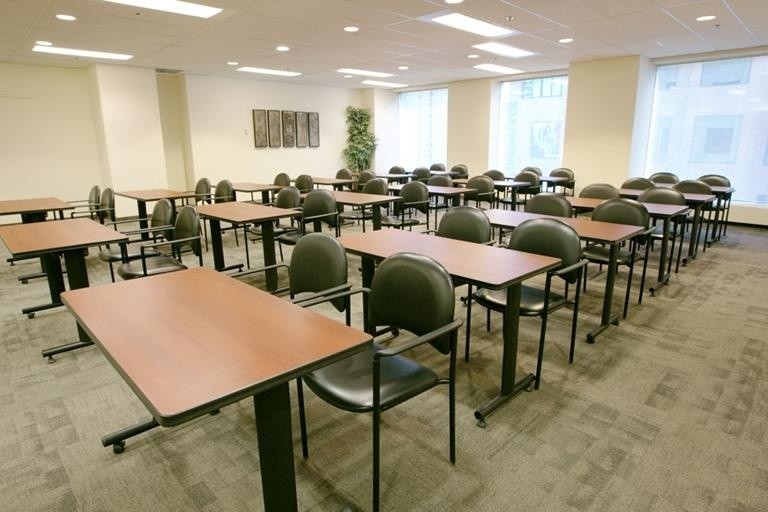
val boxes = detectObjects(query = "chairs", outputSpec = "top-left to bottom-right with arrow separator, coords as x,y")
546,168 -> 576,196
59,188 -> 120,251
200,180 -> 239,252
649,172 -> 679,184
466,218 -> 589,390
117,205 -> 203,280
275,189 -> 339,262
412,167 -> 431,216
427,174 -> 453,235
672,180 -> 712,252
53,185 -> 100,220
176,178 -> 211,236
524,193 -> 572,218
230,233 -> 352,326
521,167 -> 542,191
464,175 -> 496,210
99,199 -> 172,282
358,171 -> 375,191
337,178 -> 389,237
696,175 -> 731,241
621,177 -> 656,190
450,163 -> 469,204
629,187 -> 686,274
581,198 -> 656,319
295,175 -> 313,193
372,182 -> 431,234
500,171 -> 540,211
296,252 -> 462,512
271,173 -> 290,204
421,206 -> 496,287
430,163 -> 446,203
244,186 -> 300,269
482,170 -> 505,209
333,169 -> 352,191
388,166 -> 408,213
579,183 -> 619,199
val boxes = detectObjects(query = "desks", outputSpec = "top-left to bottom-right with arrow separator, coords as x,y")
564,196 -> 689,297
289,178 -> 359,230
176,202 -> 303,295
405,171 -> 460,209
1,218 -> 130,364
388,184 -> 479,229
452,179 -> 531,211
0,197 -> 75,223
300,191 -> 403,276
505,177 -> 568,210
654,183 -> 734,248
352,174 -> 418,216
460,209 -> 645,344
617,189 -> 716,267
113,189 -> 197,243
60,266 -> 375,511
211,183 -> 287,244
335,229 -> 562,428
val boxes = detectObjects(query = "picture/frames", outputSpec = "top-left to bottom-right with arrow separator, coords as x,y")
267,110 -> 281,147
253,109 -> 268,147
308,112 -> 319,147
296,111 -> 309,147
282,110 -> 295,147
